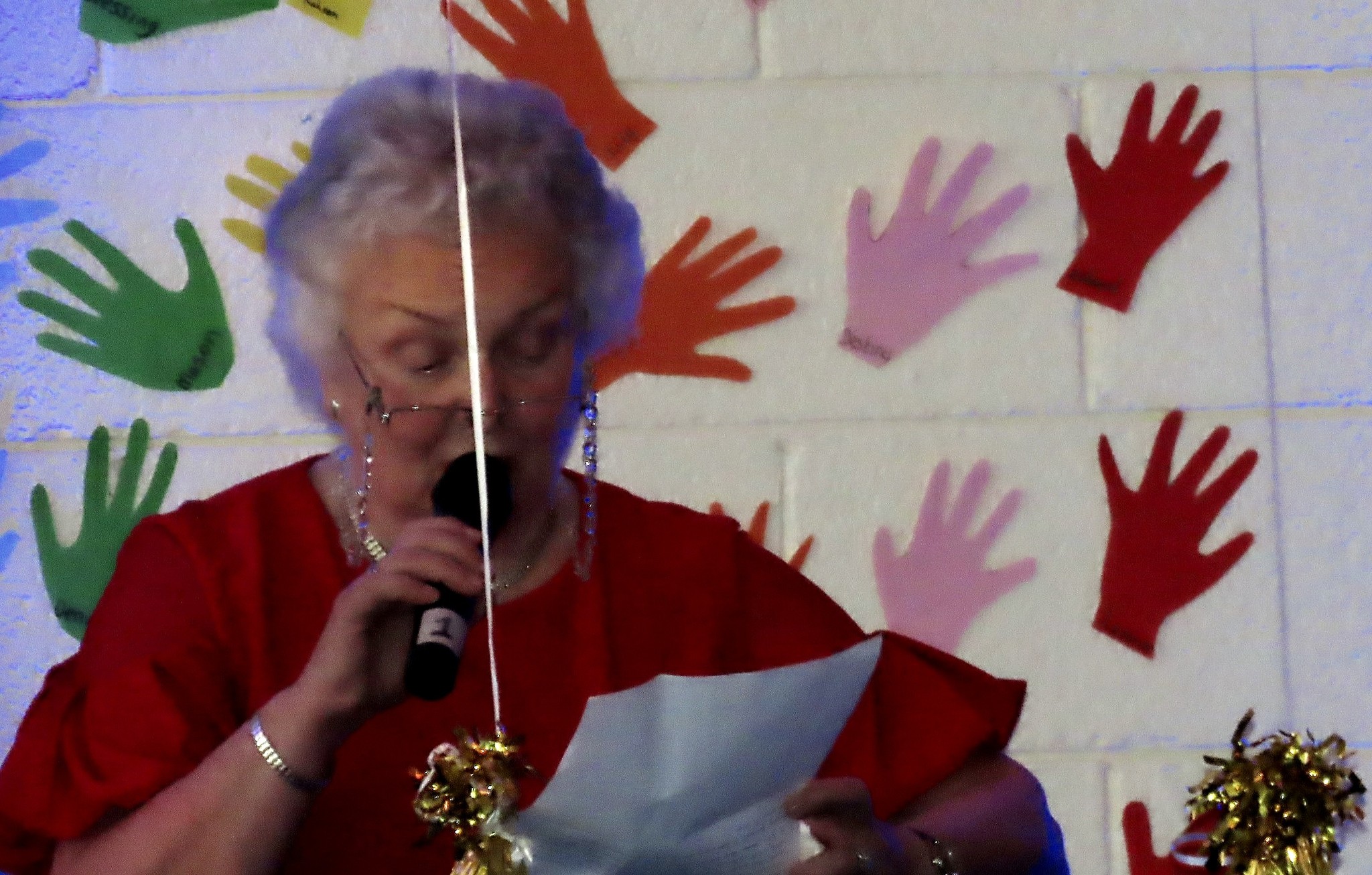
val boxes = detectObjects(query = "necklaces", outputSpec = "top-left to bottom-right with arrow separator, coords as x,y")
338,472 -> 559,594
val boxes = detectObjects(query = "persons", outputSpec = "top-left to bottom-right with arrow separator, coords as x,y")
0,77 -> 1068,875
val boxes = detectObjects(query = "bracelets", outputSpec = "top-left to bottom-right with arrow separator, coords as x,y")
907,827 -> 961,875
249,716 -> 322,794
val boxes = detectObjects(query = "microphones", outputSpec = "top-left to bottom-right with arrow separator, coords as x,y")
401,451 -> 514,703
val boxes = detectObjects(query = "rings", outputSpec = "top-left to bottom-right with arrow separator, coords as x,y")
857,852 -> 874,872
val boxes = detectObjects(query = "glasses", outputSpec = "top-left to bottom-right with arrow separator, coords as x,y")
338,331 -> 597,457
